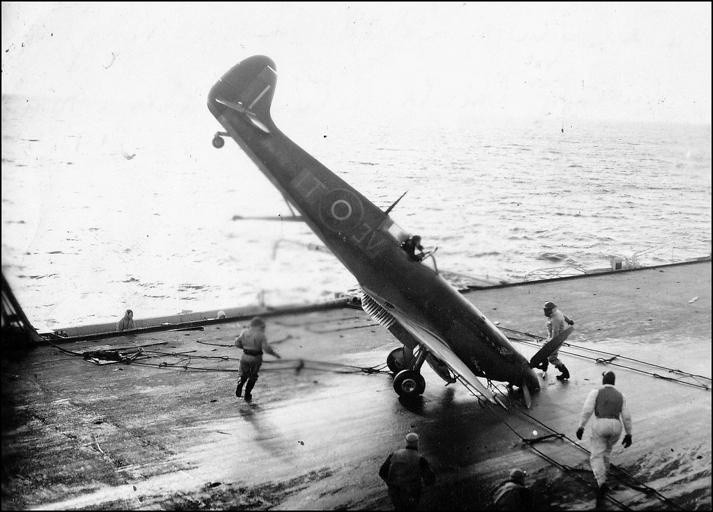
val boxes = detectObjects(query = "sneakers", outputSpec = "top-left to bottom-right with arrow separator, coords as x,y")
596,483 -> 609,496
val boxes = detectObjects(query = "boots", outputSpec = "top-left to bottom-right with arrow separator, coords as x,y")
555,363 -> 570,381
235,375 -> 259,402
536,358 -> 549,372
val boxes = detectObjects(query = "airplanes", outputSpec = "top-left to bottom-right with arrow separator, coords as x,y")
207,54 -> 573,407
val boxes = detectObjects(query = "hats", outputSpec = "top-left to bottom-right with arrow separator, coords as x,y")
544,301 -> 558,309
509,469 -> 523,480
405,432 -> 418,443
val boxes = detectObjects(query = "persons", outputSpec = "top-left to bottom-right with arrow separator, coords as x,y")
376,431 -> 434,511
539,301 -> 574,382
233,316 -> 281,404
116,309 -> 136,331
402,234 -> 425,263
488,467 -> 531,511
217,310 -> 225,319
575,370 -> 634,503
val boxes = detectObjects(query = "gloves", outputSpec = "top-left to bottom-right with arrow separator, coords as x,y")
567,319 -> 574,326
576,427 -> 585,440
621,433 -> 632,449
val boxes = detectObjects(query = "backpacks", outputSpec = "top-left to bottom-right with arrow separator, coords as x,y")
387,451 -> 421,505
